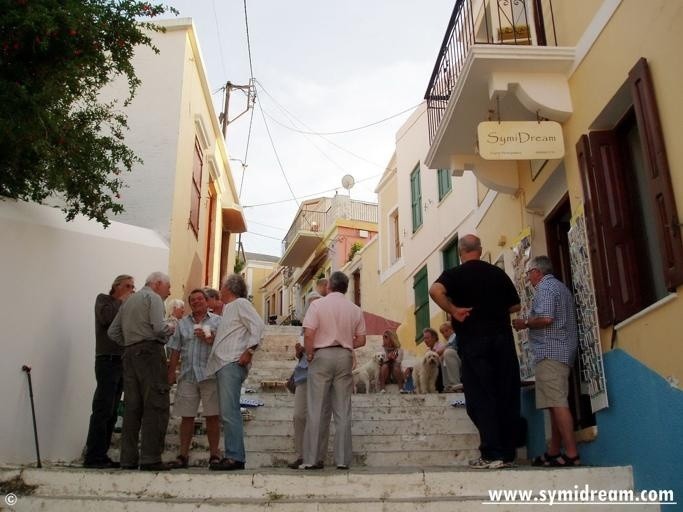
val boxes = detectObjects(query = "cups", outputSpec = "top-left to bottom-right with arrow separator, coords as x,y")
201,325 -> 211,338
193,324 -> 201,337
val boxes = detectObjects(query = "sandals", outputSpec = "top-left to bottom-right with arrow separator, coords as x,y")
542,454 -> 581,468
532,451 -> 560,465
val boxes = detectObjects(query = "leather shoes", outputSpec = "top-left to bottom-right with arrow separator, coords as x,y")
298,463 -> 324,470
83,454 -> 245,471
289,459 -> 301,470
335,463 -> 350,470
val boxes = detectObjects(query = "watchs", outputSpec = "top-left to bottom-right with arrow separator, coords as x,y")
524,319 -> 528,326
306,354 -> 312,360
247,348 -> 255,355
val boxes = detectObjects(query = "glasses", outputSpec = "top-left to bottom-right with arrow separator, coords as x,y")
525,268 -> 537,276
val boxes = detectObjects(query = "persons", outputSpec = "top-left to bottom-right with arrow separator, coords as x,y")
380,330 -> 405,394
429,233 -> 521,469
400,312 -> 462,395
304,276 -> 328,320
167,290 -> 220,468
82,274 -> 134,469
299,271 -> 367,470
205,287 -> 223,316
205,274 -> 265,470
511,255 -> 582,467
108,271 -> 176,471
165,299 -> 185,359
288,294 -> 324,468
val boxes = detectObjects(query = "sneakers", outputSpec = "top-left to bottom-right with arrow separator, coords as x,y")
468,456 -> 505,469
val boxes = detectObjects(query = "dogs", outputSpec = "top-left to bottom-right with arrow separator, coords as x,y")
411,350 -> 440,395
352,352 -> 385,394
